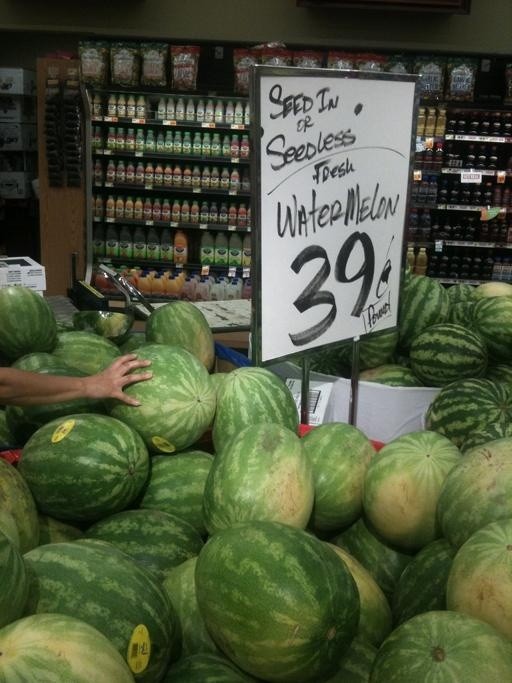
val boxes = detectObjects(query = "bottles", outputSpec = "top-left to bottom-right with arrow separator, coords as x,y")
92,94 -> 252,301
405,106 -> 512,281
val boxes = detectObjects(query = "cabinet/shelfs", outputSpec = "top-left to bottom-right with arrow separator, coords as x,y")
88,96 -> 512,301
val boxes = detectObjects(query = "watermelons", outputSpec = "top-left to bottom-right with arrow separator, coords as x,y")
1,274 -> 512,681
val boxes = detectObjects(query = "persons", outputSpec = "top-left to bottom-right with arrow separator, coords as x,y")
0,354 -> 153,407
